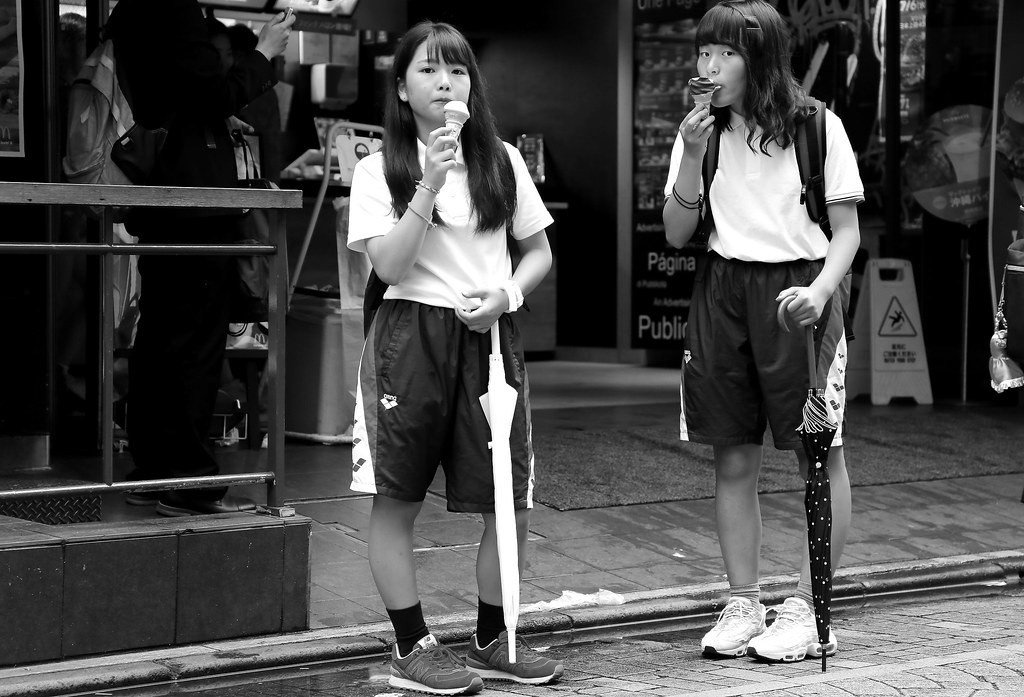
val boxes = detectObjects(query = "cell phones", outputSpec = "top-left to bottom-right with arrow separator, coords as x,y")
282,7 -> 294,22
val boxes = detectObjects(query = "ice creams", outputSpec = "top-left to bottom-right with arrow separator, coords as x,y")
688,76 -> 716,121
443,100 -> 469,151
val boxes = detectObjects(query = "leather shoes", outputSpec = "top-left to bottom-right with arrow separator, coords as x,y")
156,496 -> 257,517
127,494 -> 158,505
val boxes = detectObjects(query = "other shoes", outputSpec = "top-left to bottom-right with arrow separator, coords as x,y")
214,426 -> 239,447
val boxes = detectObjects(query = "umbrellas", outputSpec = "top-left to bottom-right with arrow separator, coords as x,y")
478,319 -> 518,663
777,295 -> 839,674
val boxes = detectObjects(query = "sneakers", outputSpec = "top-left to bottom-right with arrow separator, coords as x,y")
747,597 -> 838,662
701,595 -> 769,657
466,630 -> 564,684
388,634 -> 483,695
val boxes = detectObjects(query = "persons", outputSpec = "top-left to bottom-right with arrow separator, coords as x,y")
663,0 -> 864,663
103,0 -> 296,517
348,21 -> 564,695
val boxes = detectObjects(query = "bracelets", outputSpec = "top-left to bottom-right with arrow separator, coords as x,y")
672,183 -> 702,209
419,180 -> 440,194
500,279 -> 524,313
408,202 -> 437,230
